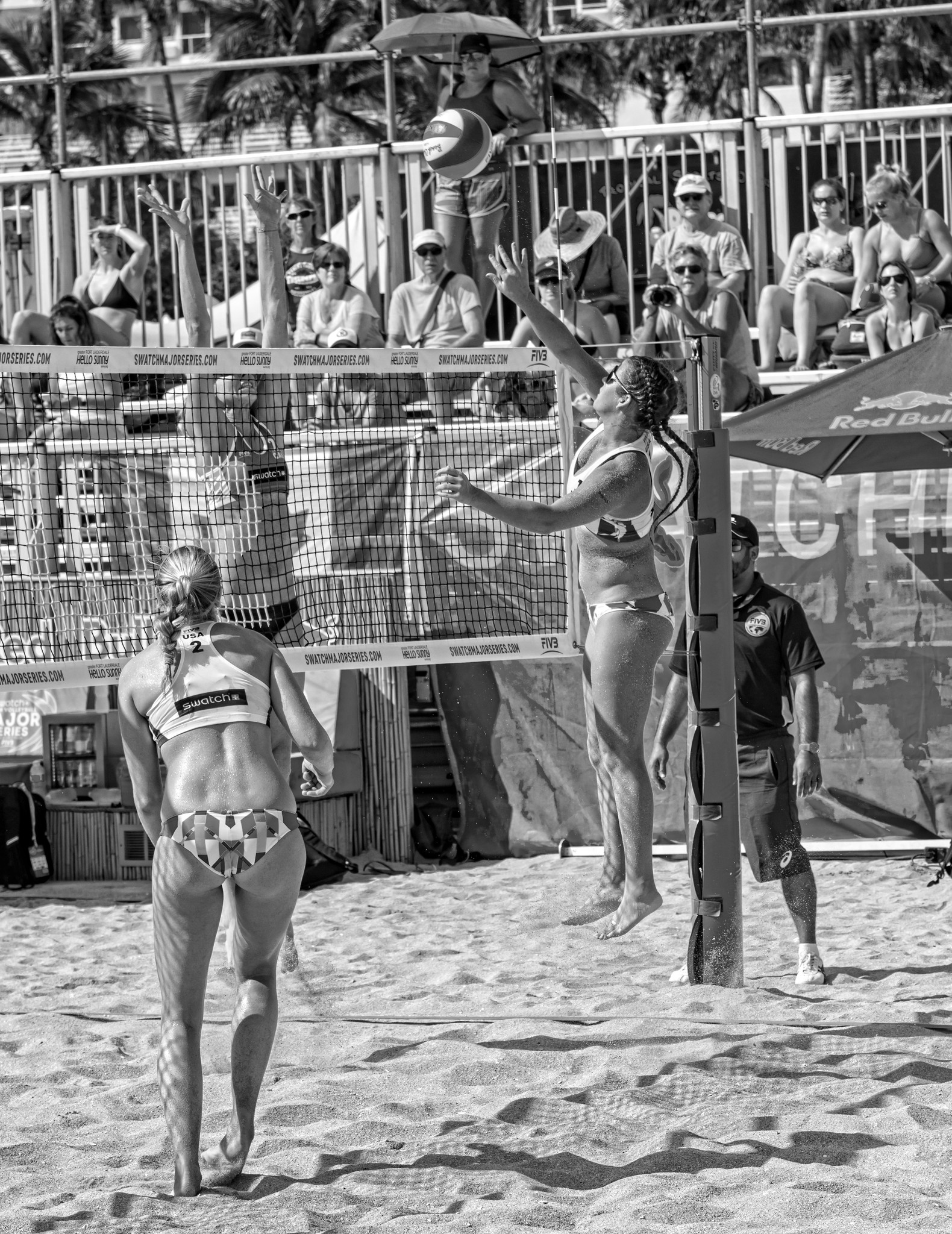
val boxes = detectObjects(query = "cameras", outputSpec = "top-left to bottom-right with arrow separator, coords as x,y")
651,289 -> 673,305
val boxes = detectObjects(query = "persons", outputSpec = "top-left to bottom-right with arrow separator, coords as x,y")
0,212 -> 152,446
212,191 -> 487,428
118,546 -> 334,1195
132,163 -> 305,973
644,514 -> 825,987
471,175 -> 762,412
754,162 -> 952,350
434,242 -> 699,939
432,28 -> 546,314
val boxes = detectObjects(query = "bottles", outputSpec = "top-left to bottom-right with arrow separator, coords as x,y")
792,256 -> 805,278
88,787 -> 122,804
46,787 -> 77,804
30,760 -> 46,798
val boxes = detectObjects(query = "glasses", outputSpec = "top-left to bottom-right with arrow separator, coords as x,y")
416,247 -> 442,256
679,193 -> 703,202
879,273 -> 910,286
812,195 -> 839,205
867,198 -> 892,212
535,276 -> 568,287
320,262 -> 345,268
606,365 -> 640,404
732,538 -> 753,552
671,264 -> 706,274
284,208 -> 316,220
459,50 -> 485,63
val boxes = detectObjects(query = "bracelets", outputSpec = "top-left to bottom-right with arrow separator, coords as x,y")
826,281 -> 837,290
256,227 -> 281,233
313,330 -> 320,345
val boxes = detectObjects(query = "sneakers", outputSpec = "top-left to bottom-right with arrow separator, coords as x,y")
668,957 -> 690,983
795,949 -> 826,985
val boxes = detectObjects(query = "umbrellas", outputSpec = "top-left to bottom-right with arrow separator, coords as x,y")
720,329 -> 952,484
364,12 -> 542,94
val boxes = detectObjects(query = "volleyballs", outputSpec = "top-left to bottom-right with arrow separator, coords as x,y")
421,109 -> 494,180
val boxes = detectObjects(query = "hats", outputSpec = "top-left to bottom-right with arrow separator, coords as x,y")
232,328 -> 263,348
731,514 -> 759,546
533,206 -> 607,263
535,255 -> 572,279
673,174 -> 713,197
412,229 -> 447,250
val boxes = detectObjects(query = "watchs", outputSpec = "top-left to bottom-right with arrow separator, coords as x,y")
923,274 -> 937,287
115,222 -> 126,236
798,741 -> 821,756
80,394 -> 88,407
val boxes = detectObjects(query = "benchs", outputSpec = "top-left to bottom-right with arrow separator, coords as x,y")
290,670 -> 362,858
121,327 -> 865,412
